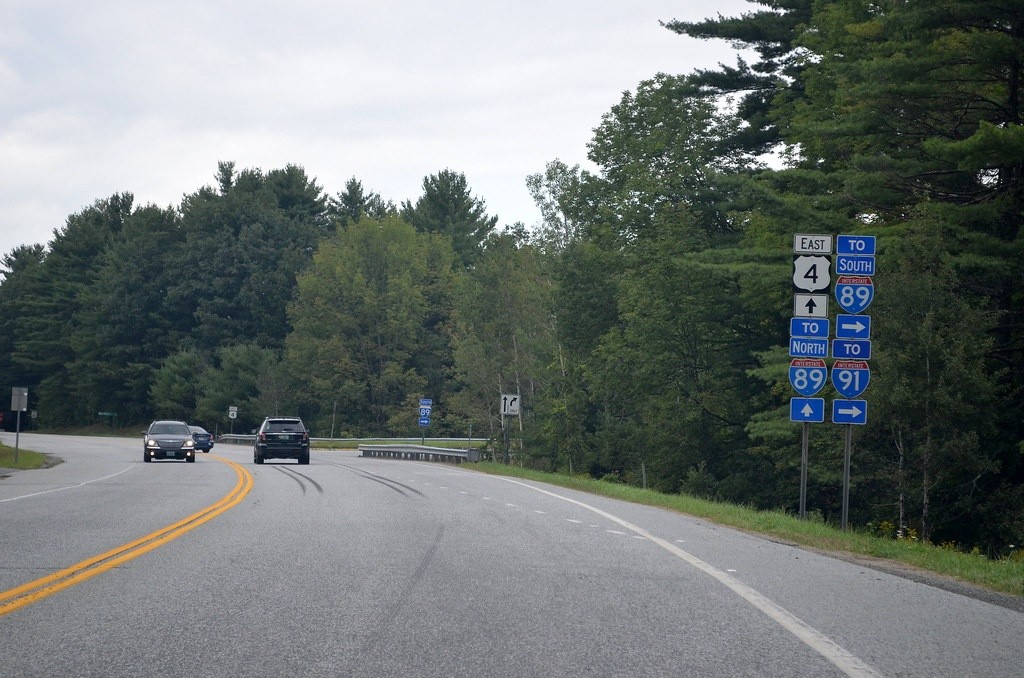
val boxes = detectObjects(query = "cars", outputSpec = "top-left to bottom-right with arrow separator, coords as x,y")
142,420 -> 196,463
187,426 -> 215,453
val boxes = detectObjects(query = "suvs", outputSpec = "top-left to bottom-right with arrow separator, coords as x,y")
252,417 -> 311,464
